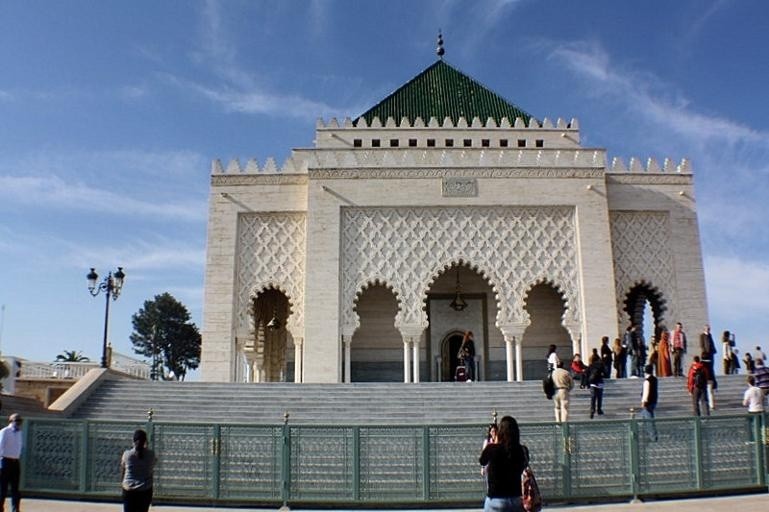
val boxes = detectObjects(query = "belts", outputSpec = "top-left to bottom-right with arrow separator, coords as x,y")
555,386 -> 569,390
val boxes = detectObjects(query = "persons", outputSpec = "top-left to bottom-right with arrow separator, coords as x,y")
479,423 -> 502,495
120,428 -> 157,511
0,412 -> 25,512
544,320 -> 768,447
458,330 -> 476,383
478,415 -> 530,511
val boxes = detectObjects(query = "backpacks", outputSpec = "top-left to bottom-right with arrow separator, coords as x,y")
691,366 -> 708,389
542,371 -> 556,399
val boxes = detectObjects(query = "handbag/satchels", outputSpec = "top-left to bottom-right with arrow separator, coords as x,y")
519,466 -> 544,511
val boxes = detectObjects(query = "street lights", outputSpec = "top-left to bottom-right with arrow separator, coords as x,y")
87,265 -> 125,372
